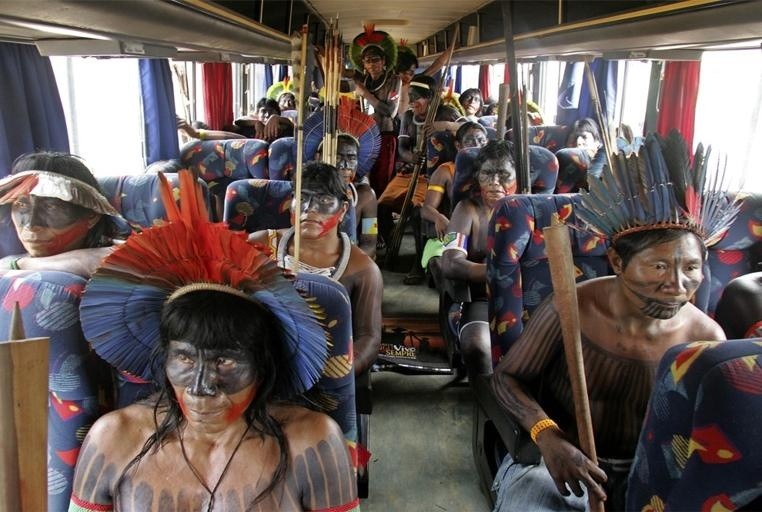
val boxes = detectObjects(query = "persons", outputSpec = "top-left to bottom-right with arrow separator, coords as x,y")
490,228 -> 727,512
0,152 -> 118,278
176,45 -> 602,292
248,161 -> 384,379
441,139 -> 518,382
68,290 -> 360,512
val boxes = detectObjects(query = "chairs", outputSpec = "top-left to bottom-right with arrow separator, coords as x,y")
0,111 -> 762,512
